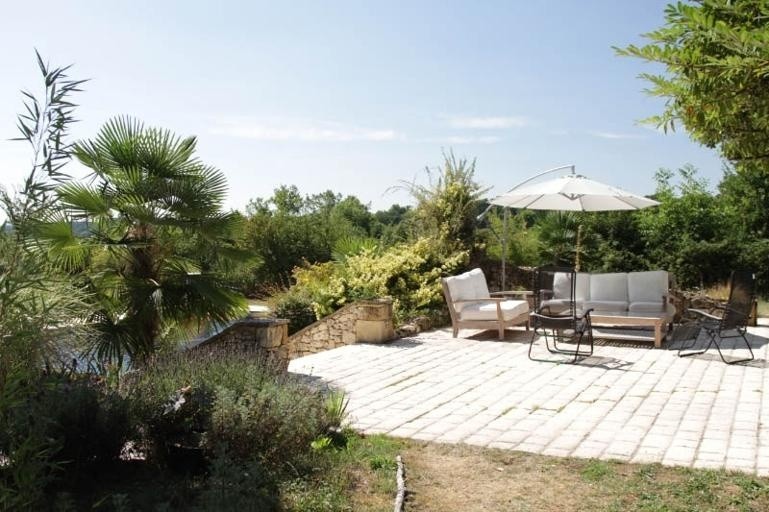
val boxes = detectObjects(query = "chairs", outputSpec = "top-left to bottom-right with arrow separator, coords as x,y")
678,269 -> 756,364
442,267 -> 530,341
528,265 -> 594,364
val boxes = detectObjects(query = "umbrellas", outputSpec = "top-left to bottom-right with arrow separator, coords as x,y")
487,163 -> 661,272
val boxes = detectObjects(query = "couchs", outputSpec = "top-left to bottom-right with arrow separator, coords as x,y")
539,270 -> 677,332
